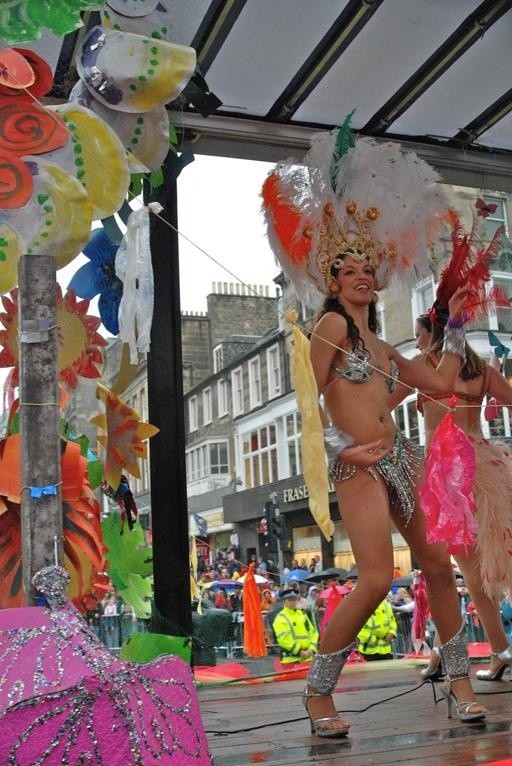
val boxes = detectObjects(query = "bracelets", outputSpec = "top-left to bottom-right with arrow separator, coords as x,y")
439,323 -> 466,363
318,424 -> 352,460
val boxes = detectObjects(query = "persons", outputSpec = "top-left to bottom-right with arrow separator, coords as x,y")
301,250 -> 488,738
394,309 -> 510,683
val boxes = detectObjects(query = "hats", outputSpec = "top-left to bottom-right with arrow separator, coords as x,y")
278,588 -> 299,599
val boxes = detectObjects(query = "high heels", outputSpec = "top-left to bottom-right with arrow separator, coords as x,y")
420,646 -> 487,722
302,684 -> 349,737
475,645 -> 512,682
